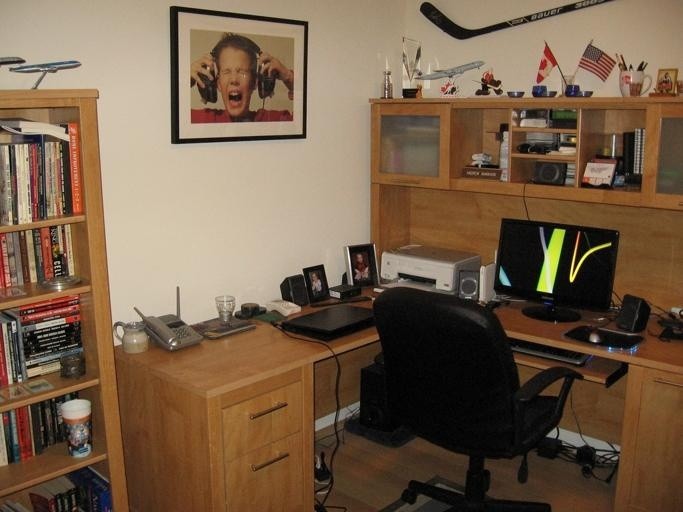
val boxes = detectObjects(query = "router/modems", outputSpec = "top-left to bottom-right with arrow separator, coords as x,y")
479,248 -> 497,304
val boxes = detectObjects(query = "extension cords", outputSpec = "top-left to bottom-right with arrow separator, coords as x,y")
314,476 -> 332,485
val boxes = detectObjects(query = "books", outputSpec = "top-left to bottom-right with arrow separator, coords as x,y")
0,466 -> 112,512
0,391 -> 79,465
0,294 -> 81,385
622,126 -> 646,187
0,224 -> 74,288
0,117 -> 82,224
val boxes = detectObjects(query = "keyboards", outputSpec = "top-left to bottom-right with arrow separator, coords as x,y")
511,340 -> 591,367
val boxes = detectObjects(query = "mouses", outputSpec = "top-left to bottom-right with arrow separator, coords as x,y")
587,330 -> 601,344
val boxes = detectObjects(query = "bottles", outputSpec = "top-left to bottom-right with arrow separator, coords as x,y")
499,131 -> 508,176
380,56 -> 394,99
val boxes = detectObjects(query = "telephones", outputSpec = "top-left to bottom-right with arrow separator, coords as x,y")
133,285 -> 204,352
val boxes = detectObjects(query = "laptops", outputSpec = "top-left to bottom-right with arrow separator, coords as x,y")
282,306 -> 376,341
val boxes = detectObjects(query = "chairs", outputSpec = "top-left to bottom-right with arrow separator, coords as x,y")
371,285 -> 584,512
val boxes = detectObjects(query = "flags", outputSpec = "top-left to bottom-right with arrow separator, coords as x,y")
578,44 -> 616,82
536,44 -> 558,84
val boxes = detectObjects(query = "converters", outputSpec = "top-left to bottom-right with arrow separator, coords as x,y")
314,467 -> 330,481
539,438 -> 560,458
576,445 -> 595,465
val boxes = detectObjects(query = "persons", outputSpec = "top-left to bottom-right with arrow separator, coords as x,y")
658,71 -> 673,94
354,254 -> 369,280
190,31 -> 294,124
312,273 -> 323,296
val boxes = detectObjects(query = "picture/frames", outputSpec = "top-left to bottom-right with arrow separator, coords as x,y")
343,242 -> 381,287
655,68 -> 678,93
303,264 -> 330,305
169,5 -> 309,144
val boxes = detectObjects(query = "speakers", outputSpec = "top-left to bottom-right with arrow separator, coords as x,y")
617,295 -> 650,332
458,268 -> 478,302
533,161 -> 568,186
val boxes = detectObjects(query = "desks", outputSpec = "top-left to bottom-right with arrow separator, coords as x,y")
114,282 -> 682,511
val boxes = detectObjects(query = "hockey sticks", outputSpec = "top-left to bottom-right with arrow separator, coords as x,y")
420,0 -> 617,39
470,78 -> 502,91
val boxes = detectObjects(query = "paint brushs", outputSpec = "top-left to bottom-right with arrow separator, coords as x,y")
616,53 -> 648,71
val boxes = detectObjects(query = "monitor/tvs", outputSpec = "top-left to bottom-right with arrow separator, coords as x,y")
493,216 -> 619,325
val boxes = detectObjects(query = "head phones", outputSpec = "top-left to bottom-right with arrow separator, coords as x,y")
197,35 -> 274,102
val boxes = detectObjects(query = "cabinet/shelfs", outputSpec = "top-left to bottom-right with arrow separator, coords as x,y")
0,88 -> 130,511
369,95 -> 683,210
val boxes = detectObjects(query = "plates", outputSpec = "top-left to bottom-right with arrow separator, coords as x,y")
506,92 -> 524,97
566,91 -> 593,98
532,91 -> 556,97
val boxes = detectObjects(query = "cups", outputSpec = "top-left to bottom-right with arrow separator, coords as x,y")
617,71 -> 653,97
562,77 -> 575,93
566,86 -> 579,97
532,86 -> 546,97
214,295 -> 233,325
61,398 -> 92,458
57,349 -> 83,380
600,134 -> 617,157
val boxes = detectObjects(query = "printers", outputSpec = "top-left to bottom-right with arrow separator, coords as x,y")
379,245 -> 480,302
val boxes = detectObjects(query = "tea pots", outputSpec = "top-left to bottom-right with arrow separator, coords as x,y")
112,322 -> 147,353
